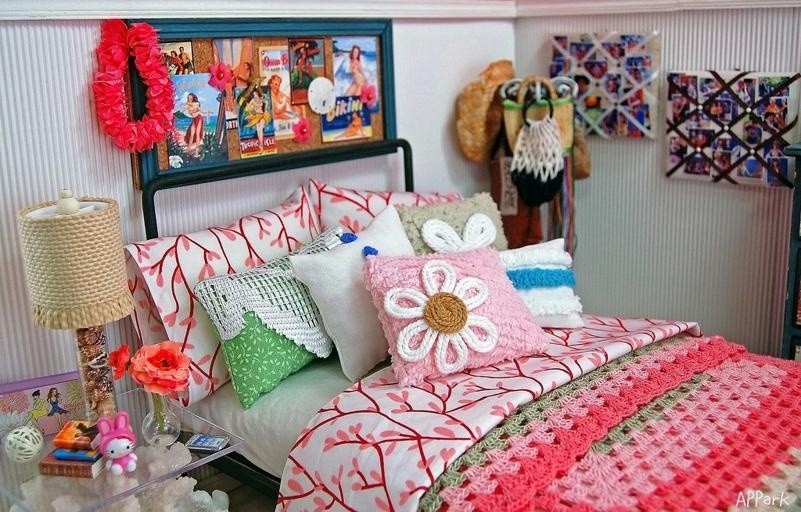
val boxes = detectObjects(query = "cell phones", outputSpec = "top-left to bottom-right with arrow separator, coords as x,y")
184,434 -> 230,451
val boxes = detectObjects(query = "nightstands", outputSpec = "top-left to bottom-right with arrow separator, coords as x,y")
0,388 -> 246,511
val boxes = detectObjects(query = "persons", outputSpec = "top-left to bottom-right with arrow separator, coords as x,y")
248,92 -> 270,152
345,46 -> 366,97
297,44 -> 310,86
182,94 -> 208,155
45,387 -> 71,432
268,74 -> 296,120
334,113 -> 364,141
23,388 -> 47,432
160,46 -> 192,74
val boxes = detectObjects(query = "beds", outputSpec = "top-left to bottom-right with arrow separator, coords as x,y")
139,136 -> 799,512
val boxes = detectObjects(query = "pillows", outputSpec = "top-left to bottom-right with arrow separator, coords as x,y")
360,246 -> 553,388
286,203 -> 416,383
112,179 -> 319,406
189,222 -> 348,411
498,236 -> 592,333
395,190 -> 512,258
305,175 -> 465,242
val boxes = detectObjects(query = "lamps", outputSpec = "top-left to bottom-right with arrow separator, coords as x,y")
14,188 -> 137,435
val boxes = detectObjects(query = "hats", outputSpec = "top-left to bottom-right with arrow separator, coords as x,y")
457,62 -> 514,161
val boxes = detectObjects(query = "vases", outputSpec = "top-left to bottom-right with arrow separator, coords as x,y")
138,394 -> 182,448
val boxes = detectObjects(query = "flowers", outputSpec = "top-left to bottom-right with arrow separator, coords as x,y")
359,84 -> 378,107
205,63 -> 236,92
91,17 -> 176,153
107,340 -> 192,395
289,117 -> 313,142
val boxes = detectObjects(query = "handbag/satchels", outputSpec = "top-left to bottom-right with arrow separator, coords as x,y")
489,77 -> 591,247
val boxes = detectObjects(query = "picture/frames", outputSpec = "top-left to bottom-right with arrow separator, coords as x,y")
0,370 -> 85,440
122,14 -> 398,190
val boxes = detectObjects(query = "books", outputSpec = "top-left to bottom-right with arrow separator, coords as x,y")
40,420 -> 112,479
